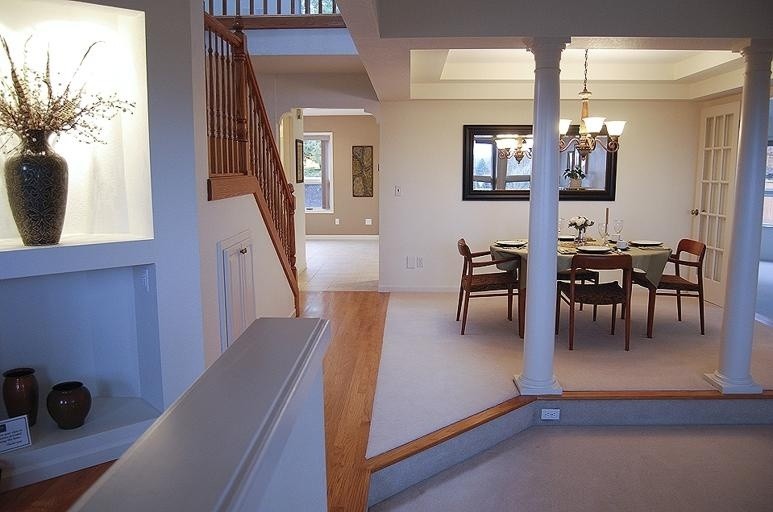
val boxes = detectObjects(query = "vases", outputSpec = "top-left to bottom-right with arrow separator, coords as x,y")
574,230 -> 588,248
3,129 -> 69,247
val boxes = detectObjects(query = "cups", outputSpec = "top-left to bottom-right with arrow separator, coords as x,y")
617,239 -> 628,249
612,234 -> 620,242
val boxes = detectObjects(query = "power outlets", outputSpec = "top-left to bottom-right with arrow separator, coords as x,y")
417,256 -> 424,267
541,409 -> 560,420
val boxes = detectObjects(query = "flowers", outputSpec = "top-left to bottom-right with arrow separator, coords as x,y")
567,216 -> 594,230
0,34 -> 140,156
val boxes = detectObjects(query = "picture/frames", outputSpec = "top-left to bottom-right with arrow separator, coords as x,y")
352,145 -> 373,197
296,139 -> 304,183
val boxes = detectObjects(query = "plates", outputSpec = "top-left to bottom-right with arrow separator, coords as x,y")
578,246 -> 610,253
497,241 -> 526,245
631,241 -> 662,245
558,236 -> 578,240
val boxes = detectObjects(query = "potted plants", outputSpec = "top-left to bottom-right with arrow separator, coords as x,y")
564,167 -> 586,188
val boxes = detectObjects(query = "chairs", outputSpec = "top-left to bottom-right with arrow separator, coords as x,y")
556,254 -> 632,351
621,239 -> 706,338
456,238 -> 520,335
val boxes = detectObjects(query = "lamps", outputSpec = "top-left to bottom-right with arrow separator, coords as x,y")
495,134 -> 532,165
559,48 -> 628,161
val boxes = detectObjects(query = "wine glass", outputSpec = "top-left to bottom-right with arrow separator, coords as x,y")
613,219 -> 623,239
598,223 -> 612,246
